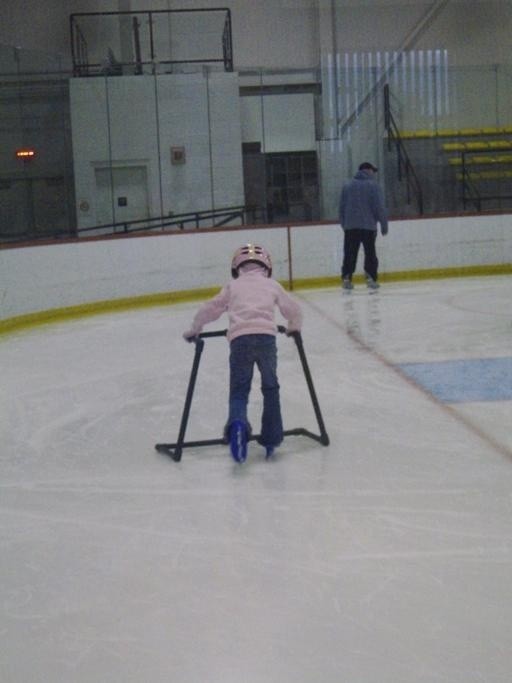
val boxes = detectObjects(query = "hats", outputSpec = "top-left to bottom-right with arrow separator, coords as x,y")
358,160 -> 380,173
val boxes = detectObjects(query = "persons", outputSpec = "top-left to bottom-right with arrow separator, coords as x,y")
184,241 -> 304,464
337,161 -> 389,287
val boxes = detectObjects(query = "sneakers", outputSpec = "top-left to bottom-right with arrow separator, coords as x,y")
228,420 -> 250,463
257,437 -> 281,458
341,277 -> 382,290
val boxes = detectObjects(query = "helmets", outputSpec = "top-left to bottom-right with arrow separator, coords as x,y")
229,241 -> 274,278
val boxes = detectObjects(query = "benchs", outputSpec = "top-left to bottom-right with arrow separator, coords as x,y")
390,128 -> 512,210
